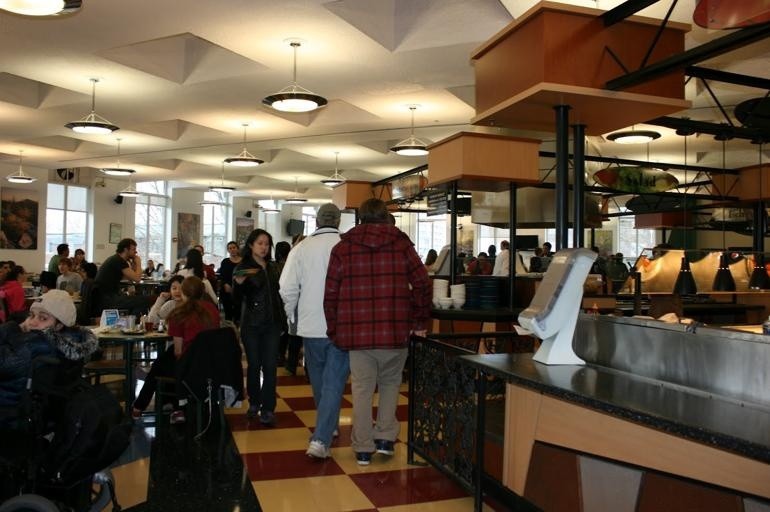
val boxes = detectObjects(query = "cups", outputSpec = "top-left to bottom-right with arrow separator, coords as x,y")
152,272 -> 159,281
144,316 -> 155,332
126,315 -> 137,330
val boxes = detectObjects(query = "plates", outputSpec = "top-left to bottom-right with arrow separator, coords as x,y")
232,269 -> 260,277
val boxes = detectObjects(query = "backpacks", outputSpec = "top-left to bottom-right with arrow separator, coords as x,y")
24,380 -> 135,482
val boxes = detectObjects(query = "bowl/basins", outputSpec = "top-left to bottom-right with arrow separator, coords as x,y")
432,279 -> 466,310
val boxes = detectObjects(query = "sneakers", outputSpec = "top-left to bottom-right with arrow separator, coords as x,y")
354,452 -> 370,465
130,400 -> 142,420
276,354 -> 310,383
375,439 -> 394,456
246,404 -> 273,423
162,403 -> 186,425
305,429 -> 339,462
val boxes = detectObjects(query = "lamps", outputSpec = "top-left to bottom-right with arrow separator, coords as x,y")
63,79 -> 120,136
284,179 -> 307,203
209,163 -> 236,193
261,46 -> 326,112
118,177 -> 141,198
222,126 -> 263,167
388,105 -> 429,157
0,0 -> 81,16
99,140 -> 135,176
256,194 -> 280,214
4,150 -> 37,184
607,125 -> 660,144
319,153 -> 346,188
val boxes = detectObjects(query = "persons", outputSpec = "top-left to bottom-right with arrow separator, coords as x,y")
1,240 -> 243,426
279,203 -> 350,459
422,241 -> 629,281
232,229 -> 284,423
268,242 -> 303,374
324,199 -> 433,465
1,288 -> 99,480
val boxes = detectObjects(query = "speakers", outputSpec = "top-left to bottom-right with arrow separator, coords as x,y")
116,195 -> 123,203
247,211 -> 251,217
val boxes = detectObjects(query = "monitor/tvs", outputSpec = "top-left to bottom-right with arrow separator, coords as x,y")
287,218 -> 305,237
512,247 -> 600,366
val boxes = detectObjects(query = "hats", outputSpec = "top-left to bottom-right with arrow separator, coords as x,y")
30,289 -> 77,327
318,203 -> 340,220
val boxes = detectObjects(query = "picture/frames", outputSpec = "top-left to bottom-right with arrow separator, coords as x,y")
177,212 -> 200,258
0,186 -> 39,250
236,217 -> 254,249
109,223 -> 121,243
